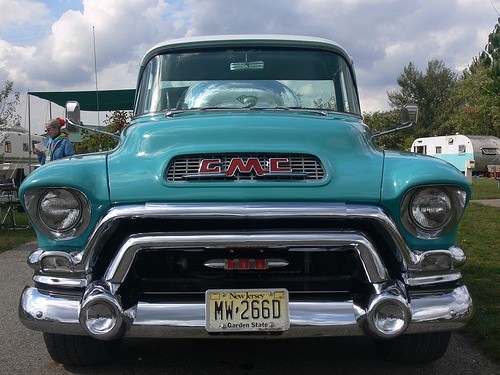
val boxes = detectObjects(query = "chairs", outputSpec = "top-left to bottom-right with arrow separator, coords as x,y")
2,179 -> 33,230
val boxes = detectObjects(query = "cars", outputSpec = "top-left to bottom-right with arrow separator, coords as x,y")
19,33 -> 473,364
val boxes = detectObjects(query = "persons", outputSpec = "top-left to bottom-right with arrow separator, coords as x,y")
32,117 -> 75,165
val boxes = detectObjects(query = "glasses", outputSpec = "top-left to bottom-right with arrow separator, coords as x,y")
45,129 -> 53,132
